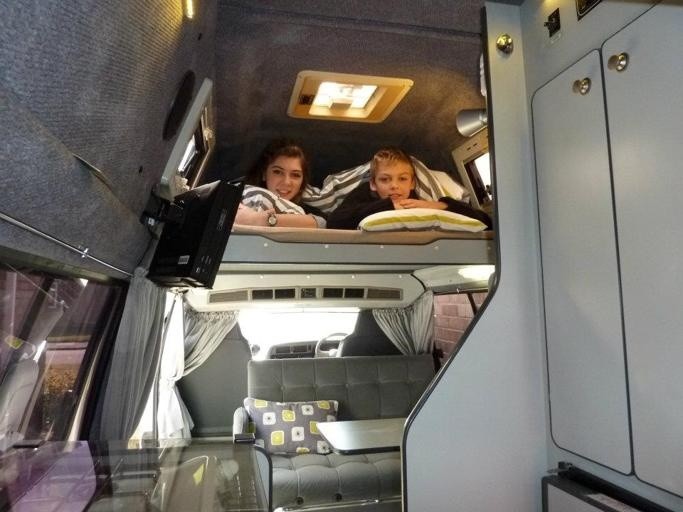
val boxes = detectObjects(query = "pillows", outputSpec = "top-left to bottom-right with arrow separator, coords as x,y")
244,397 -> 339,454
358,208 -> 488,233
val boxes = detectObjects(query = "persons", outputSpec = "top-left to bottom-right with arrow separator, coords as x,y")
328,145 -> 492,230
233,136 -> 328,229
337,310 -> 401,356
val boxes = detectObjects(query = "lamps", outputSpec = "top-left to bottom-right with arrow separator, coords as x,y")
456,109 -> 487,138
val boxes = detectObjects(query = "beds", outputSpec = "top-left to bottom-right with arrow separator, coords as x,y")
183,223 -> 495,313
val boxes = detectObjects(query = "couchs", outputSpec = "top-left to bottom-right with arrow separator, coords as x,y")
232,355 -> 436,512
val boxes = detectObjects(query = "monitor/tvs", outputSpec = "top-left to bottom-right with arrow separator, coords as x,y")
144,174 -> 248,291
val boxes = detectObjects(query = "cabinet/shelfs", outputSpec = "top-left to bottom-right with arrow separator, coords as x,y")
531,4 -> 683,500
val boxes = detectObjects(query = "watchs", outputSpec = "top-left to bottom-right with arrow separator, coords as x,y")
268,213 -> 277,227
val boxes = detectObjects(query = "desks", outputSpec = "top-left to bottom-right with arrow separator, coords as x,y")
0,437 -> 272,512
